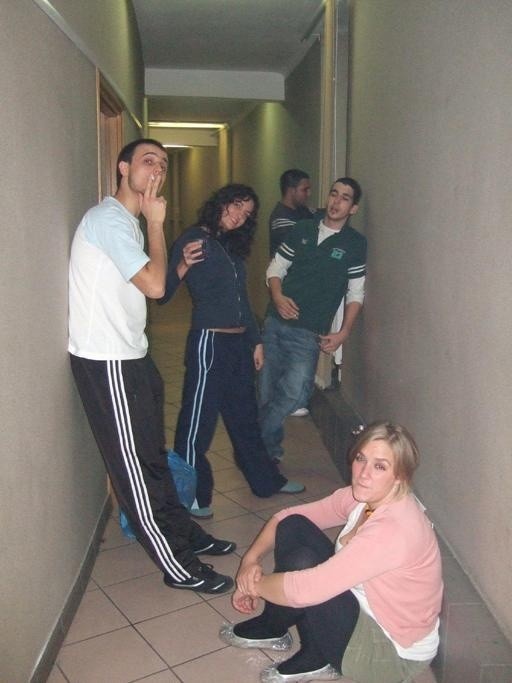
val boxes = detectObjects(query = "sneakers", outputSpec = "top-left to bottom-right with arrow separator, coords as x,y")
260,664 -> 340,683
164,563 -> 234,594
218,621 -> 292,651
188,507 -> 214,520
289,407 -> 308,417
276,480 -> 305,493
191,535 -> 238,556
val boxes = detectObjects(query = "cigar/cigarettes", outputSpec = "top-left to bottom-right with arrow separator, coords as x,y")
149,174 -> 155,183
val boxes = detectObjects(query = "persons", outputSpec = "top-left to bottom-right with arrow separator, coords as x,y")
268,165 -> 315,261
258,175 -> 369,462
67,138 -> 237,598
216,420 -> 445,682
157,182 -> 308,521
